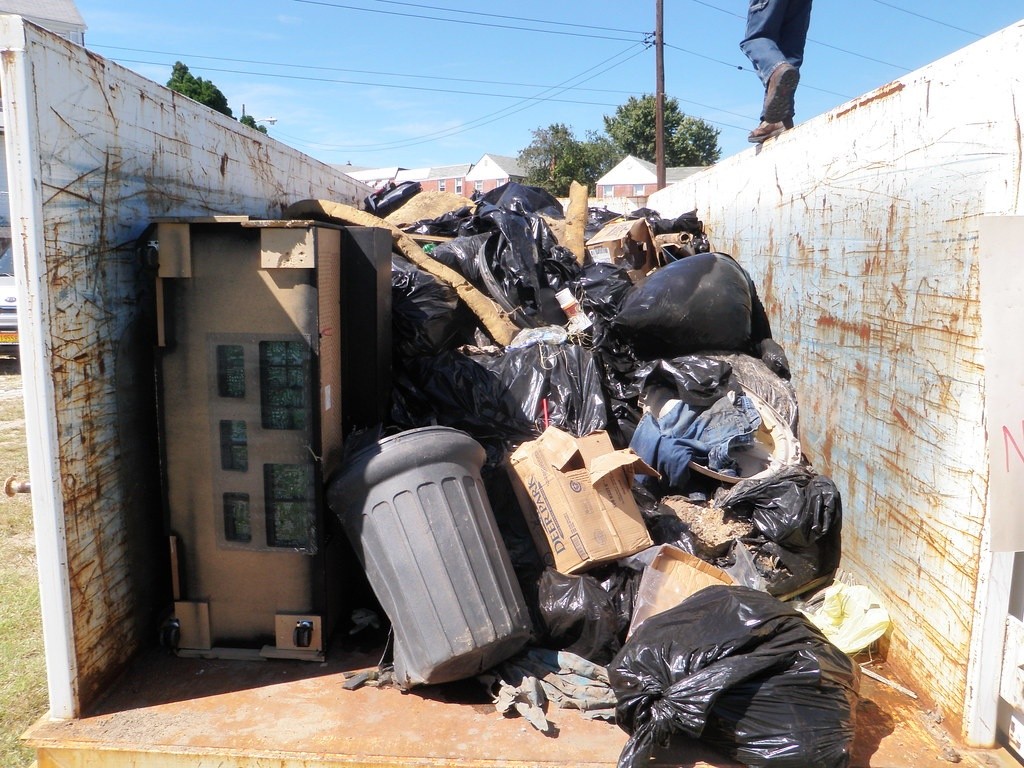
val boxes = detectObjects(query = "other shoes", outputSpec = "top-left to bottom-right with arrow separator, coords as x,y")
762,62 -> 802,125
747,117 -> 793,143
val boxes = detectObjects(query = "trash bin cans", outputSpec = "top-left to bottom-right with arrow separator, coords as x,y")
317,421 -> 539,689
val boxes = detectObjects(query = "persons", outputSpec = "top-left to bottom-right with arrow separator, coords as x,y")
738,0 -> 813,141
